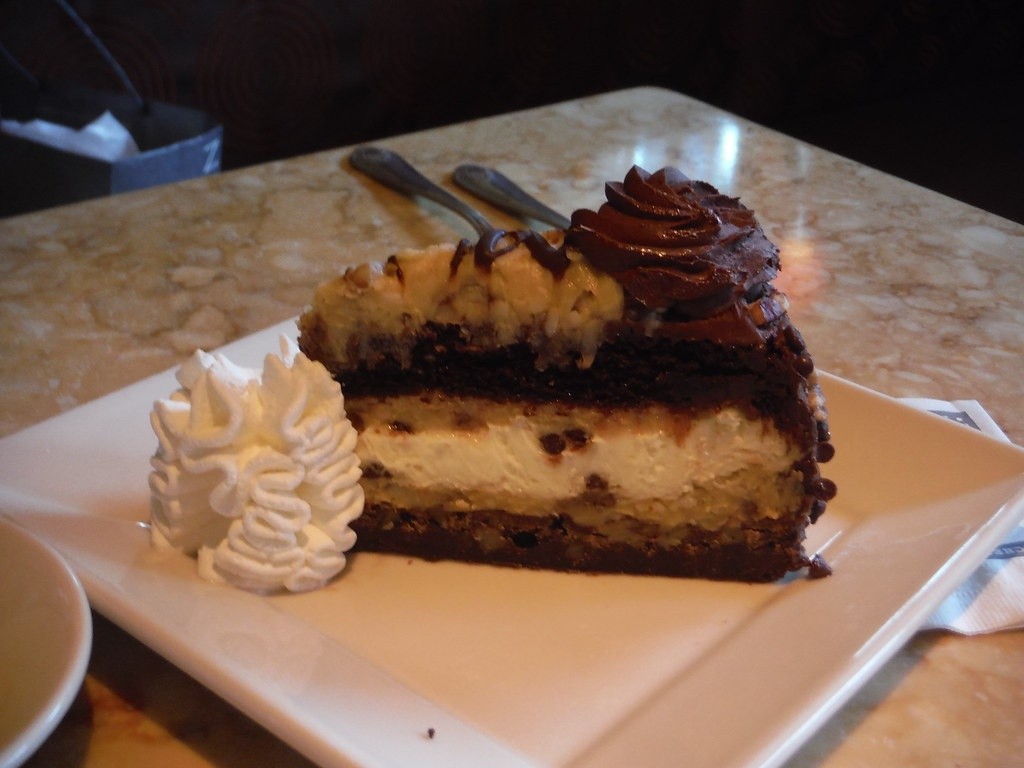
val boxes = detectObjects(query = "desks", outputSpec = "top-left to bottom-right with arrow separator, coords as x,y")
0,85 -> 1024,768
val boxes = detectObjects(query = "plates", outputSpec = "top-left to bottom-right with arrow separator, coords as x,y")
1,323 -> 1024,768
0,517 -> 94,768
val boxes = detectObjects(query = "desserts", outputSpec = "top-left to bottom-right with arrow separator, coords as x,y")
147,163 -> 838,595
